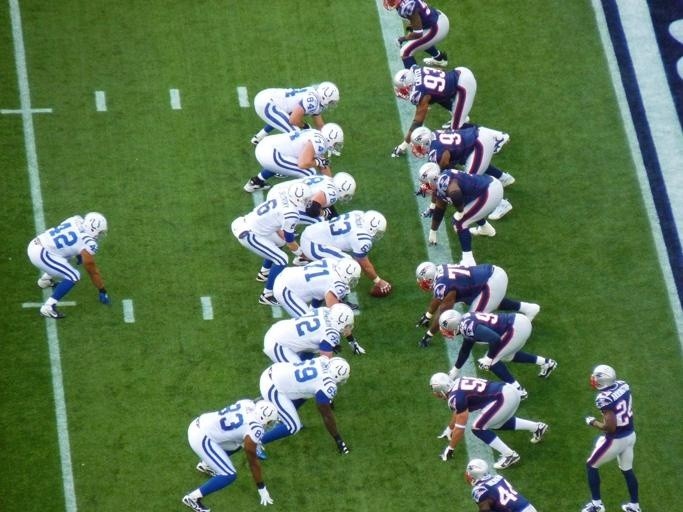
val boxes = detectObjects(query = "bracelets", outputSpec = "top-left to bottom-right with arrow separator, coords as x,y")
291,247 -> 302,256
371,276 -> 383,286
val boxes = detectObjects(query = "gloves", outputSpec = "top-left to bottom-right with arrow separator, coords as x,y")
479,358 -> 493,372
415,312 -> 433,346
437,426 -> 454,460
76,255 -> 83,263
99,287 -> 109,303
334,436 -> 348,454
420,204 -> 438,246
391,142 -> 408,157
258,486 -> 273,505
346,336 -> 366,355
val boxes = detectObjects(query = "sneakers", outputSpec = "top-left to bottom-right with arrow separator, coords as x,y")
293,257 -> 310,265
502,173 -> 514,187
517,385 -> 528,400
38,278 -> 61,289
182,462 -> 215,511
441,118 -> 452,129
257,444 -> 269,460
469,197 -> 512,238
582,499 -> 641,511
424,53 -> 448,67
256,269 -> 280,306
243,177 -> 271,192
525,303 -> 540,321
492,132 -> 510,154
495,422 -> 547,468
40,304 -> 65,318
537,357 -> 557,378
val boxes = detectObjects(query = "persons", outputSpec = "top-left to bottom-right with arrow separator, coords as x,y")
249,80 -> 340,146
261,303 -> 354,365
266,170 -> 357,242
229,182 -> 315,306
273,257 -> 367,355
26,211 -> 112,319
242,123 -> 345,192
181,399 -> 279,512
581,364 -> 642,512
385,1 -> 558,512
255,354 -> 352,456
292,209 -> 391,294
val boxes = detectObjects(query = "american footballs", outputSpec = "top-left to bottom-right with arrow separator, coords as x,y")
371,282 -> 391,297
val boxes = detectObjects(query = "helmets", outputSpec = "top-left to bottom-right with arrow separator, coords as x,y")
415,262 -> 437,291
330,303 -> 354,335
328,357 -> 350,385
437,310 -> 461,337
466,459 -> 491,484
323,123 -> 344,156
410,127 -> 441,191
591,364 -> 617,391
289,171 -> 356,207
318,80 -> 341,108
394,70 -> 414,99
256,400 -> 279,430
84,212 -> 108,239
338,259 -> 361,287
363,210 -> 387,240
429,372 -> 455,399
